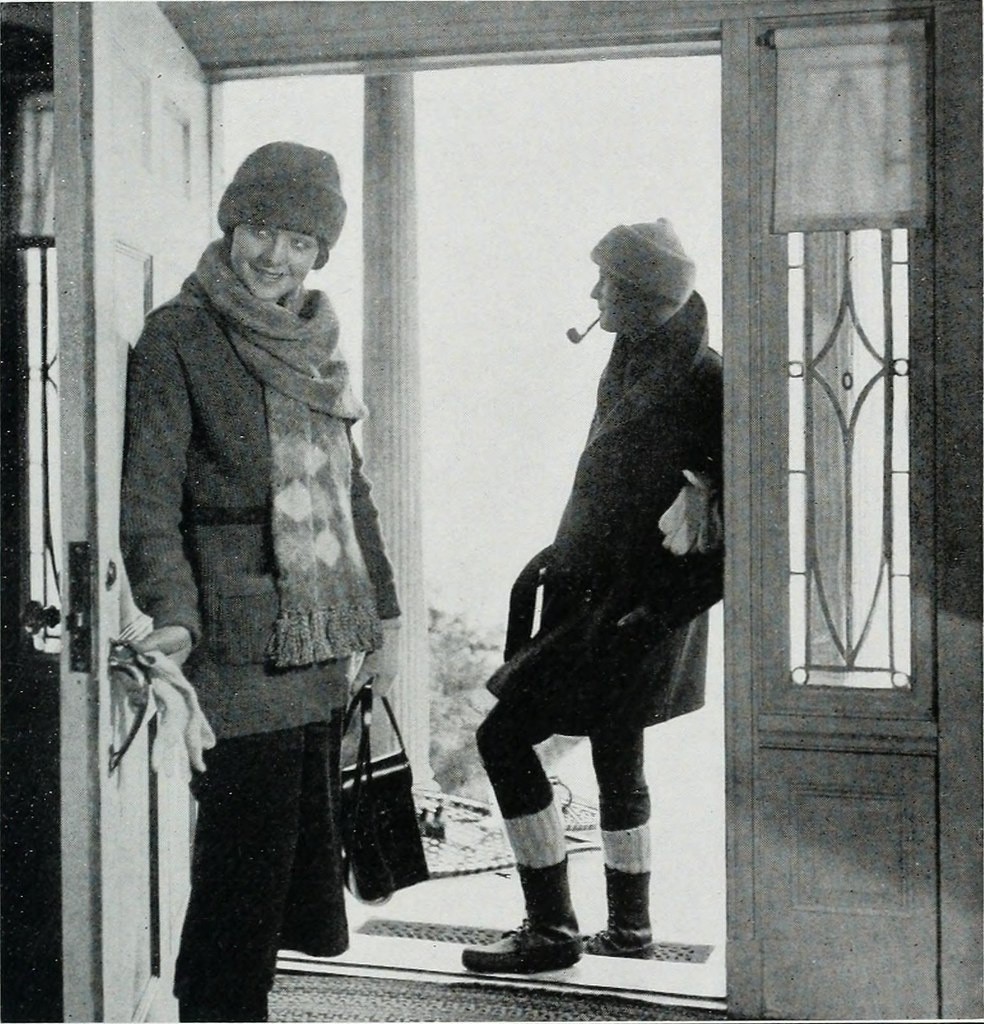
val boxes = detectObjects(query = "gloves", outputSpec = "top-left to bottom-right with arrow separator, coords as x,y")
112,650 -> 216,785
658,471 -> 725,557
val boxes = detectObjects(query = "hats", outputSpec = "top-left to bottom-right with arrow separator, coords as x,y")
590,215 -> 698,305
217,141 -> 349,269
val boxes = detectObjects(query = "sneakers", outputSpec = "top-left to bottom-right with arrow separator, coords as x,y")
583,927 -> 657,958
461,907 -> 586,975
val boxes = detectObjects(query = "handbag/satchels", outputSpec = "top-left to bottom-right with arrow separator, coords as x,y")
337,689 -> 432,902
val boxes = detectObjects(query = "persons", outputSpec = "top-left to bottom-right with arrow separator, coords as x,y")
119,143 -> 403,1023
463,219 -> 724,975
